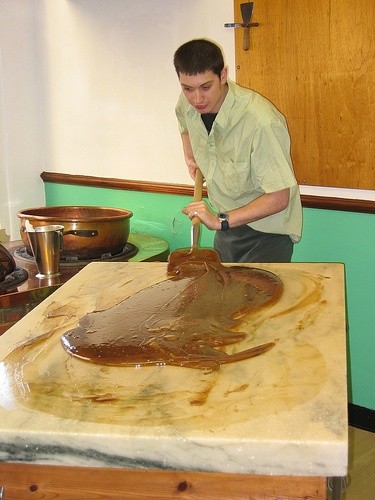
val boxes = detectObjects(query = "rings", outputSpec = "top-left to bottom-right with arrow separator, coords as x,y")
195,210 -> 198,217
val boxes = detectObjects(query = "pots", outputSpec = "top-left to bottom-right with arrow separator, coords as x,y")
16,206 -> 133,261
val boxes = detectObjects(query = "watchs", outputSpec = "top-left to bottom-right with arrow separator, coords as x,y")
216,211 -> 231,231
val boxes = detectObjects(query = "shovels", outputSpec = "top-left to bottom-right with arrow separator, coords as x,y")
166,166 -> 222,277
239,1 -> 253,51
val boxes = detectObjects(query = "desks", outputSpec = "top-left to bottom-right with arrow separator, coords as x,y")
0,260 -> 348,500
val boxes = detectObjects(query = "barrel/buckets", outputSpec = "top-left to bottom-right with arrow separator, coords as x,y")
26,225 -> 64,279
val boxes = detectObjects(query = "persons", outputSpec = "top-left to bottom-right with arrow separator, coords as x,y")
174,38 -> 304,264
